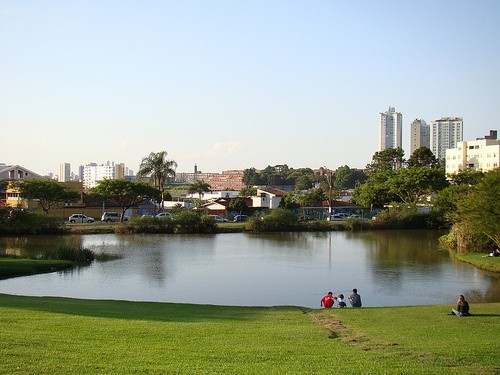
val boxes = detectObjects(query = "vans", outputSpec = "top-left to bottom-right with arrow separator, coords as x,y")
154,213 -> 171,219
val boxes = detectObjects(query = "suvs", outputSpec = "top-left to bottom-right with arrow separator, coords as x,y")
207,215 -> 229,222
234,215 -> 250,222
101,212 -> 130,224
69,213 -> 95,223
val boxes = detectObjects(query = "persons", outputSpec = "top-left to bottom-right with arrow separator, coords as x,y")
481,248 -> 500,258
320,292 -> 347,308
348,289 -> 362,307
447,294 -> 469,317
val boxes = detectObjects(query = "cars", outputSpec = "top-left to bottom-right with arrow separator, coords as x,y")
326,213 -> 360,222
